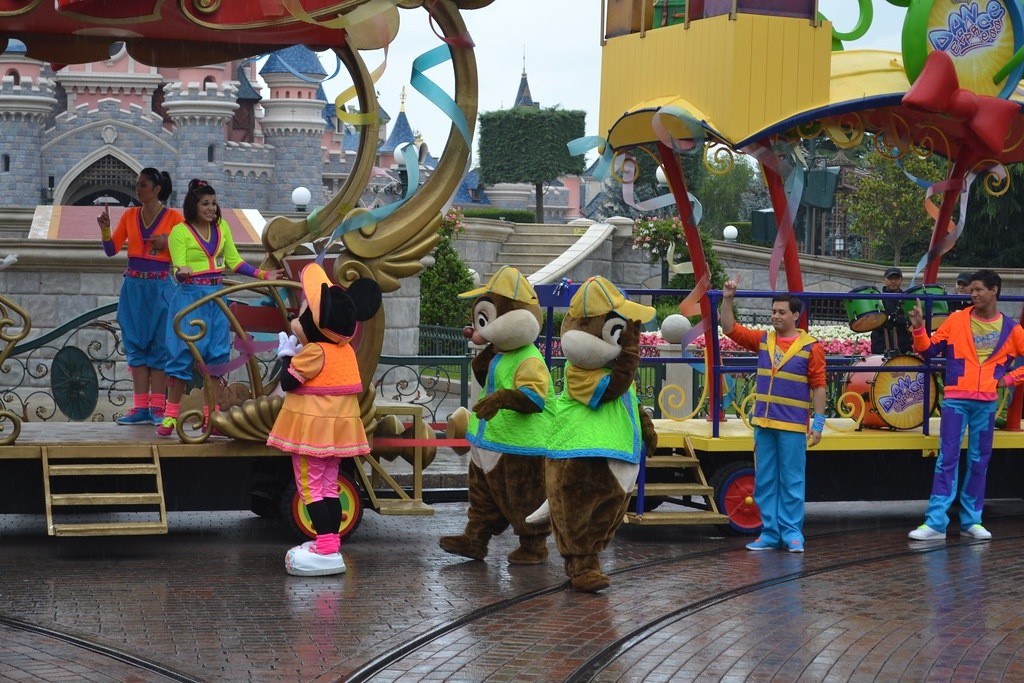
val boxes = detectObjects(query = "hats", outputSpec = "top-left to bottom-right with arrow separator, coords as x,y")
957,272 -> 973,285
885,268 -> 902,278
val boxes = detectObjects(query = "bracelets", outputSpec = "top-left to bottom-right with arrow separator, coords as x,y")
811,413 -> 826,433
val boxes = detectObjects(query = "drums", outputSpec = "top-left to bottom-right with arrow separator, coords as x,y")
841,354 -> 940,430
898,283 -> 951,333
843,285 -> 888,334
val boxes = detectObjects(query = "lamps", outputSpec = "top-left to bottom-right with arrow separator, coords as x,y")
722,226 -> 738,243
292,186 -> 313,212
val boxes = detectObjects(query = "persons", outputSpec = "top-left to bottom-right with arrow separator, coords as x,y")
941,272 -> 975,385
721,272 -> 827,552
871,269 -> 912,354
97,168 -> 184,425
155,178 -> 284,437
907,270 -> 1024,539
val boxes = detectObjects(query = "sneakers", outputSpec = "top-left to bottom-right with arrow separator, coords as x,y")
960,524 -> 992,539
745,539 -> 776,550
149,407 -> 165,425
156,417 -> 177,436
202,425 -> 227,438
116,408 -> 151,424
908,525 -> 946,540
782,540 -> 804,552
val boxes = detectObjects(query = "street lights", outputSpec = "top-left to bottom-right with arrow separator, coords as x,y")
657,163 -> 672,289
394,142 -> 419,202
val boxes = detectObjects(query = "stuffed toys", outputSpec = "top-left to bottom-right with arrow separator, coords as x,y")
545,276 -> 656,589
266,262 -> 383,577
439,264 -> 556,564
